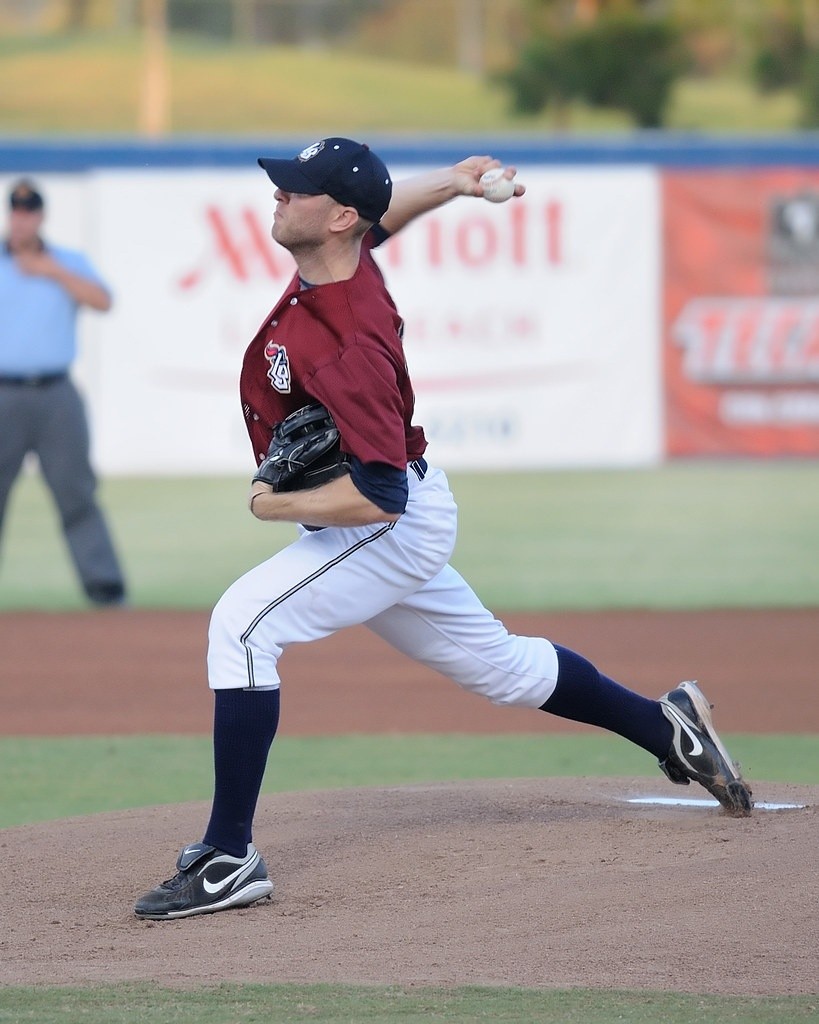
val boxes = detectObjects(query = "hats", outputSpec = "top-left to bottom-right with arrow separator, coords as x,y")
258,137 -> 392,223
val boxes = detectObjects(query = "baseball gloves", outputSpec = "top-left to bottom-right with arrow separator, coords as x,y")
250,405 -> 353,533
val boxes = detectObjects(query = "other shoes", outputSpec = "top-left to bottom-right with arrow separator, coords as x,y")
88,581 -> 121,601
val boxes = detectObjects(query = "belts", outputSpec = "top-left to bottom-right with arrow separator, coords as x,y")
300,455 -> 430,532
0,372 -> 67,388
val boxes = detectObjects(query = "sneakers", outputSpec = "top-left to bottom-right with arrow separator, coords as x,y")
134,843 -> 274,920
657,680 -> 755,814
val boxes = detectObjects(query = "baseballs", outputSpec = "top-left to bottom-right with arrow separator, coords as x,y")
479,167 -> 515,204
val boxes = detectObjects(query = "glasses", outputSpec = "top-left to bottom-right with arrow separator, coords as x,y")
11,194 -> 41,210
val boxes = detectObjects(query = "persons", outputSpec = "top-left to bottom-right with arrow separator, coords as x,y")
0,178 -> 127,604
132,135 -> 754,922
